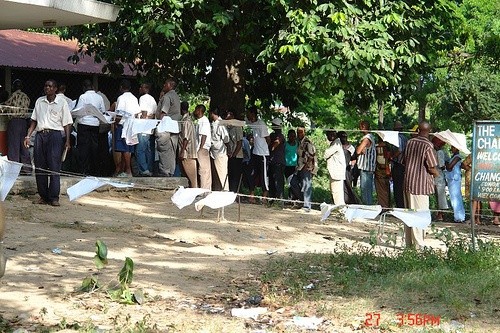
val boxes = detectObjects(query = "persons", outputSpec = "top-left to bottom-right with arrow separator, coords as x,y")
24,78 -> 73,206
0,76 -> 500,237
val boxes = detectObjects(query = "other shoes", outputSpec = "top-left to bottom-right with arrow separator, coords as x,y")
51,200 -> 60,206
241,198 -> 256,204
32,198 -> 50,204
152,172 -> 170,177
301,207 -> 311,212
291,203 -> 304,210
110,171 -> 134,178
134,172 -> 152,177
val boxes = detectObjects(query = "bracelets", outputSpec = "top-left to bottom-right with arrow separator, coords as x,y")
24,137 -> 30,139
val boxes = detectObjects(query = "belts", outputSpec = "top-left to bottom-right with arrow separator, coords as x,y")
37,129 -> 58,134
